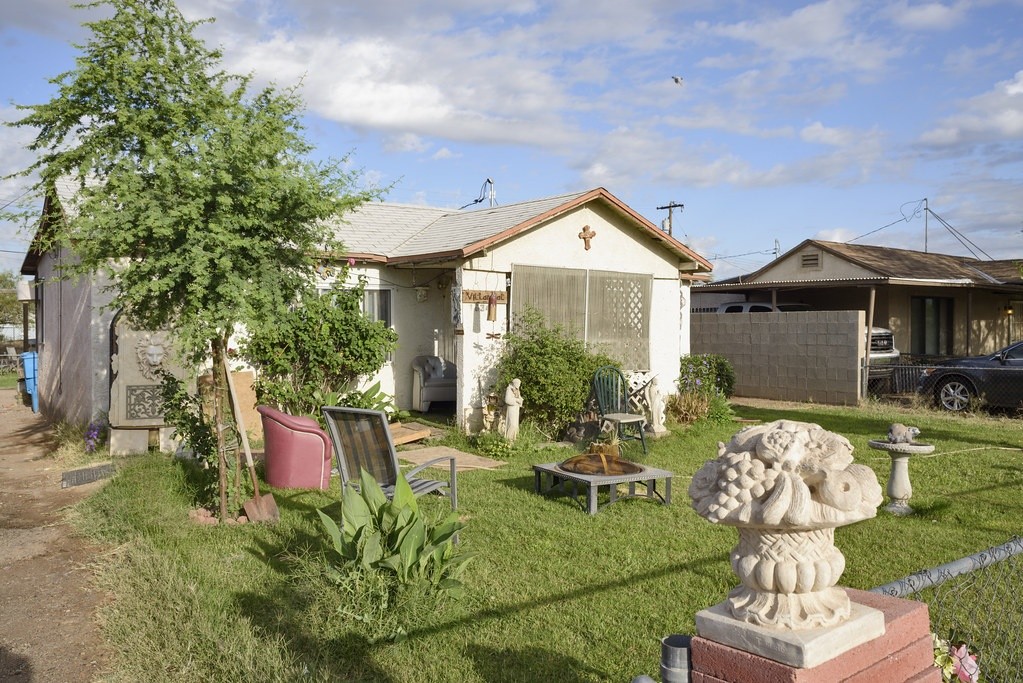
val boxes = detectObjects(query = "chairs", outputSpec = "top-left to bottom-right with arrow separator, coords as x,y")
257,405 -> 333,490
321,406 -> 460,545
593,366 -> 648,456
412,356 -> 457,412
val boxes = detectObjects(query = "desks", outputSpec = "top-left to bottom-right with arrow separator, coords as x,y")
531,455 -> 674,514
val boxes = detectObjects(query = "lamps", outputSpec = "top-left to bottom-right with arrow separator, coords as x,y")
1006,305 -> 1014,315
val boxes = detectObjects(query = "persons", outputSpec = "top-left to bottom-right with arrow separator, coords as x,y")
504,377 -> 523,441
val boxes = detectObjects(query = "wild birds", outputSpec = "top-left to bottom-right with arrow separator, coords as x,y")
671,76 -> 683,85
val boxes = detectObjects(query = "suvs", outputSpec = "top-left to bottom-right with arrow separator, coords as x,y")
716,301 -> 900,393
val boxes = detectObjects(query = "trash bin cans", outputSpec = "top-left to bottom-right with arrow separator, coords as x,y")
22,352 -> 39,414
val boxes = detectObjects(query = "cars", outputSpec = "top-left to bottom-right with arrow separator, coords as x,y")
917,341 -> 1023,416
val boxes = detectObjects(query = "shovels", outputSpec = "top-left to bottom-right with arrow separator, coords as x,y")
221,347 -> 278,522
